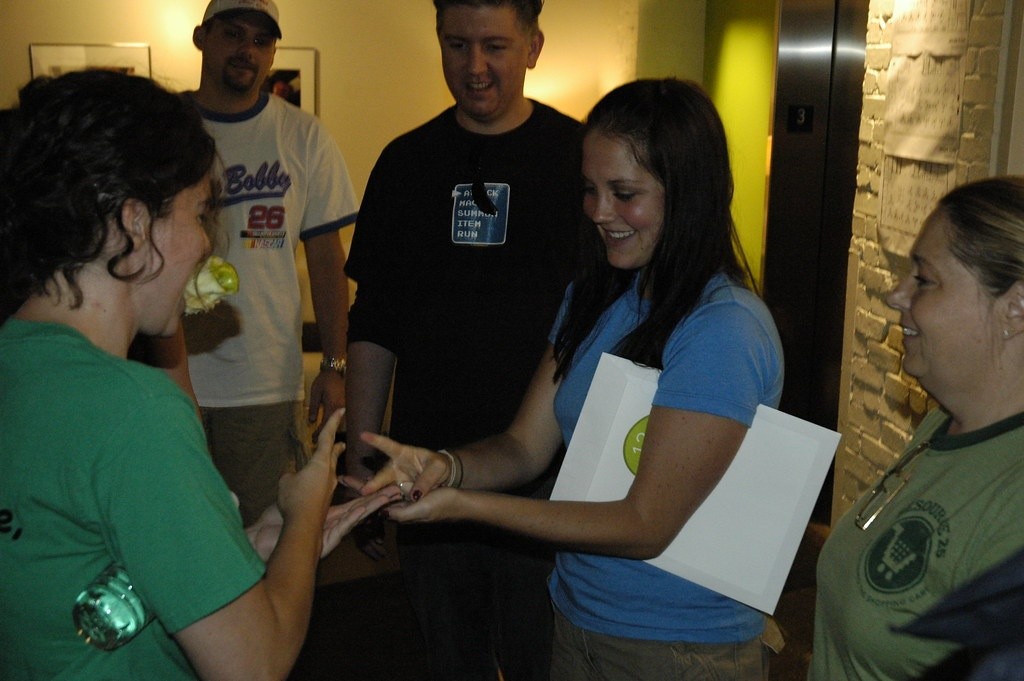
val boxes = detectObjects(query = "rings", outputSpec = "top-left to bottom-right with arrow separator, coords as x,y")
399,479 -> 412,489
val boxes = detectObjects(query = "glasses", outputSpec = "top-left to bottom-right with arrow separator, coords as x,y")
854,441 -> 933,530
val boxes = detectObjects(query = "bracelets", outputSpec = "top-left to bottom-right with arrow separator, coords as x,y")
320,356 -> 347,373
437,449 -> 464,489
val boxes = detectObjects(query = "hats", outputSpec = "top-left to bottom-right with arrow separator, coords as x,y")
201,0 -> 283,41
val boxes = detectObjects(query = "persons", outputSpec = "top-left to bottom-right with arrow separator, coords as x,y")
179,1 -> 348,527
342,1 -> 594,681
1,65 -> 348,681
361,73 -> 787,680
805,175 -> 1024,681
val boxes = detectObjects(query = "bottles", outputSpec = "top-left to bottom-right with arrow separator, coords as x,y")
70,489 -> 241,651
